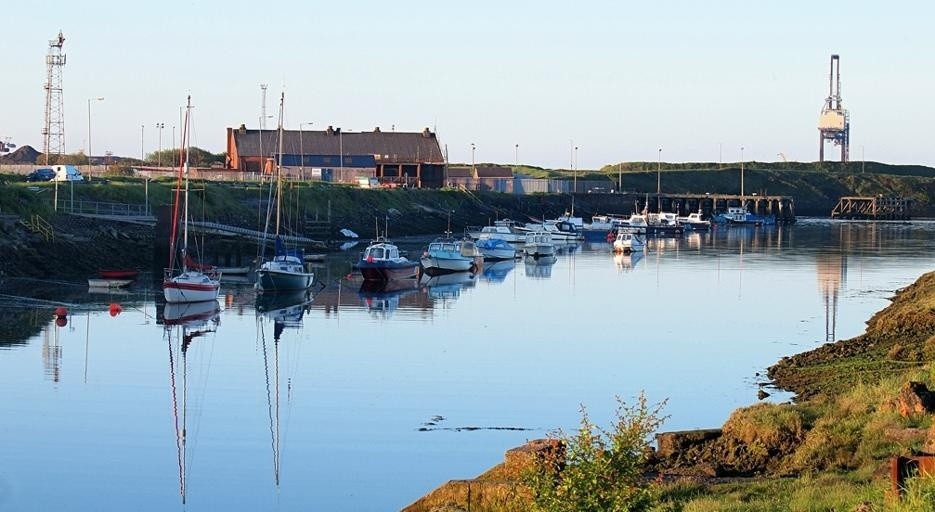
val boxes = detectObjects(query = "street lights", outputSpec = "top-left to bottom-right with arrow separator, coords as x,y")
339,129 -> 354,182
141,125 -> 145,168
86,94 -> 106,186
658,148 -> 662,195
514,144 -> 519,176
574,147 -> 577,194
740,146 -> 746,197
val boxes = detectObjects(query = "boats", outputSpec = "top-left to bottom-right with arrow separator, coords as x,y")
86,269 -> 140,289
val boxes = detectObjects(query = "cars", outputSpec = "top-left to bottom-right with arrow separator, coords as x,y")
26,168 -> 55,181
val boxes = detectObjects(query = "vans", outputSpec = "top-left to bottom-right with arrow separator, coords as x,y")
52,164 -> 83,181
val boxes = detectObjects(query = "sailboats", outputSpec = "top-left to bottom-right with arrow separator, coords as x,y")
160,82 -> 317,303
160,293 -> 315,504
